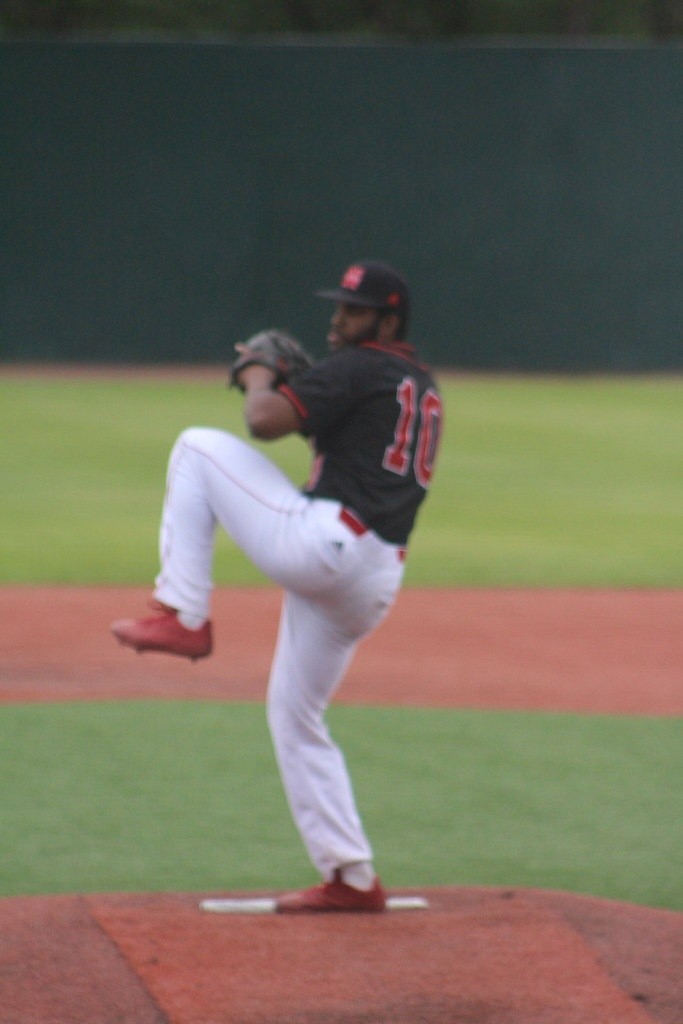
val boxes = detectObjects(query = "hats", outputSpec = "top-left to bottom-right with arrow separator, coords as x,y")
313,262 -> 408,308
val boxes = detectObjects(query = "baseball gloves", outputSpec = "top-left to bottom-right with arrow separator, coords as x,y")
230,332 -> 317,395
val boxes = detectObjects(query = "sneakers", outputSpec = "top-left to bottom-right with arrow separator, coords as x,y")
274,868 -> 384,914
110,601 -> 212,662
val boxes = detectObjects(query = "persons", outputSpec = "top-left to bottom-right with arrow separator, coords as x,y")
116,266 -> 445,910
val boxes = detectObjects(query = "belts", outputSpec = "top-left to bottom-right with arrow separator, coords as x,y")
341,507 -> 408,562
